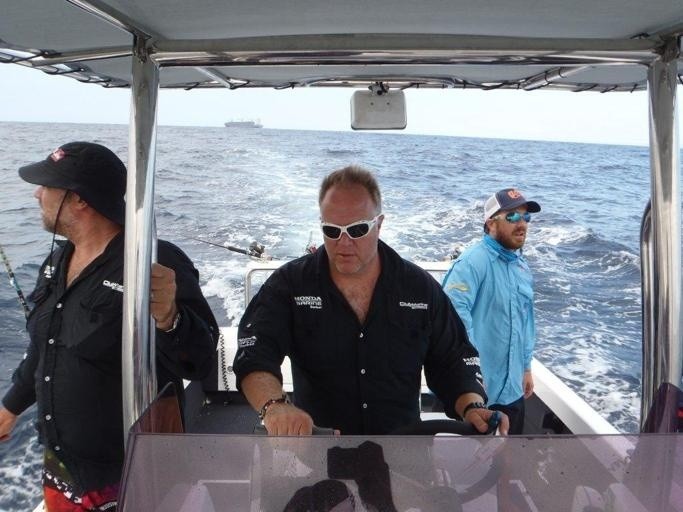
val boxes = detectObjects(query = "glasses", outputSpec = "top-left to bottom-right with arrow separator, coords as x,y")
319,212 -> 384,241
493,211 -> 531,223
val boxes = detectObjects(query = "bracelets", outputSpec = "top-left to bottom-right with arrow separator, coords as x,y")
164,311 -> 182,336
258,393 -> 294,422
463,401 -> 489,417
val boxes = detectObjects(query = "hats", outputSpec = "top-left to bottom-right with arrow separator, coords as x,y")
484,188 -> 541,233
18,142 -> 127,226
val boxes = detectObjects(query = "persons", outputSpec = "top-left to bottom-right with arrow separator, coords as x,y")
441,187 -> 540,435
232,161 -> 511,437
0,141 -> 220,512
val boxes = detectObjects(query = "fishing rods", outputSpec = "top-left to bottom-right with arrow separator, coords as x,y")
177,232 -> 284,261
